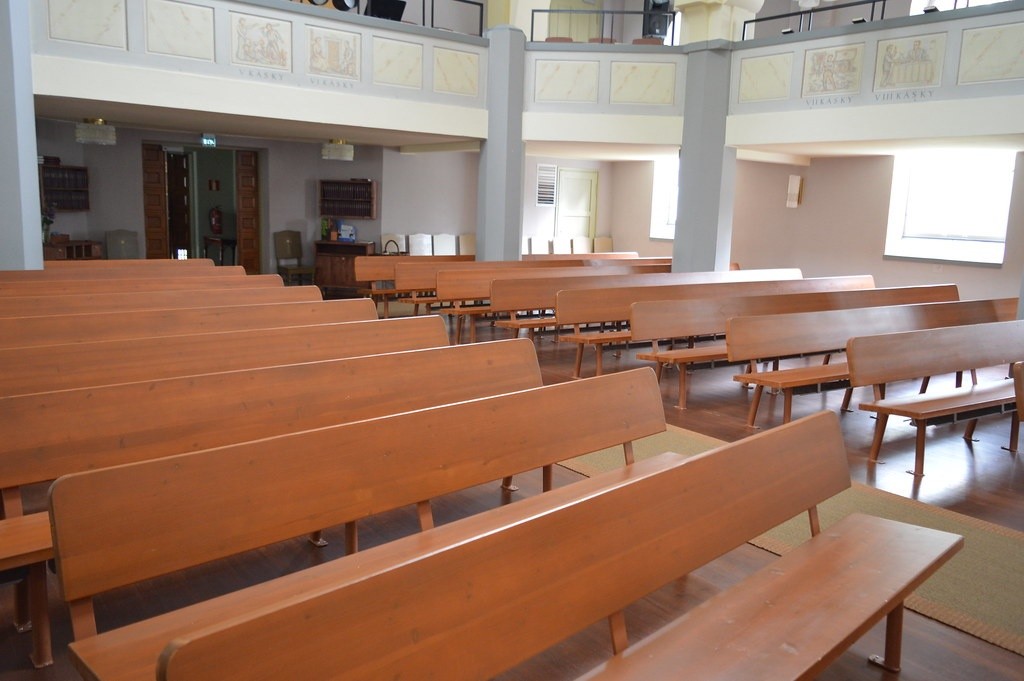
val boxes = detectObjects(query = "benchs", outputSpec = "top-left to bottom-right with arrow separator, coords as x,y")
731,263 -> 741,271
0,314 -> 451,631
48,367 -> 697,681
0,285 -> 323,324
355,252 -> 639,319
846,319 -> 1024,477
156,411 -> 966,681
437,264 -> 671,345
395,257 -> 673,315
0,274 -> 284,297
629,283 -> 959,409
0,266 -> 246,284
0,297 -> 379,352
44,258 -> 214,271
557,275 -> 876,378
490,268 -> 803,358
0,338 -> 551,667
726,296 -> 1020,428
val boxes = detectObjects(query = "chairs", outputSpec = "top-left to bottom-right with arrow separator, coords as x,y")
522,236 -> 528,254
531,236 -> 549,254
573,236 -> 592,254
380,233 -> 406,299
409,234 -> 432,297
274,230 -> 316,286
459,234 -> 478,254
364,0 -> 406,22
106,228 -> 139,260
594,236 -> 613,253
553,237 -> 571,254
433,233 -> 457,255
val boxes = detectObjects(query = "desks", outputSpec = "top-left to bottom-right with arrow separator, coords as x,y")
204,235 -> 237,266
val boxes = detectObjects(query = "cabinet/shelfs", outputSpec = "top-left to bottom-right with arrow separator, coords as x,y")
319,179 -> 377,220
43,240 -> 101,260
314,239 -> 376,290
39,164 -> 90,213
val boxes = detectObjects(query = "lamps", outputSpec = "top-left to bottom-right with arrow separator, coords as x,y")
308,0 -> 359,12
321,138 -> 354,162
75,118 -> 116,146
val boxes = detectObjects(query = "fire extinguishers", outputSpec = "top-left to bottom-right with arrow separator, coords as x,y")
209,205 -> 223,235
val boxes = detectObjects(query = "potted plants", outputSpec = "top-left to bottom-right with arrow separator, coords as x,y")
42,202 -> 59,240
